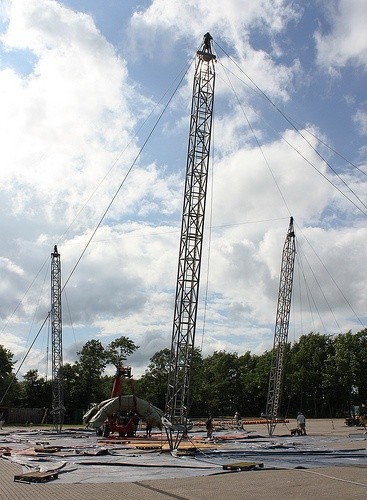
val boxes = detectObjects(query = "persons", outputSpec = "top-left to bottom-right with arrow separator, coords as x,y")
297,412 -> 307,436
205,416 -> 215,439
233,411 -> 244,431
146,422 -> 153,436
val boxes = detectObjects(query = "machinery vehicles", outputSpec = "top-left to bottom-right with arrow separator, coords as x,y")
89,364 -> 140,437
